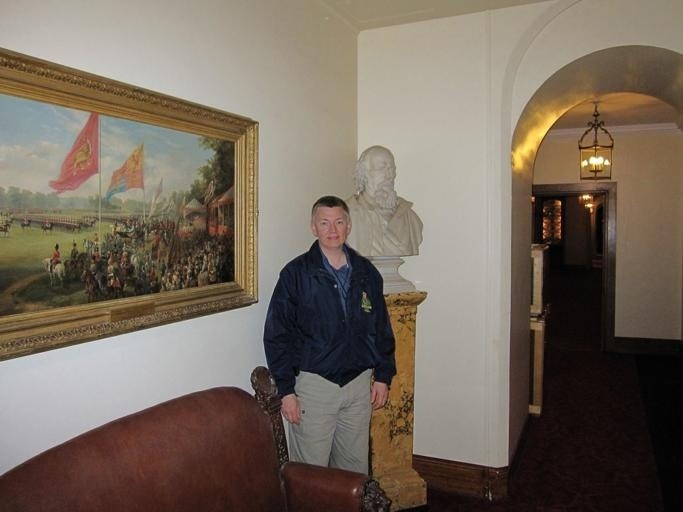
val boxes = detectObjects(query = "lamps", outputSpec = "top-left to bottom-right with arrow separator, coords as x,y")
573,119 -> 617,182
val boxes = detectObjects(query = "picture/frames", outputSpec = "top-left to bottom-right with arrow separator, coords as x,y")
0,43 -> 260,361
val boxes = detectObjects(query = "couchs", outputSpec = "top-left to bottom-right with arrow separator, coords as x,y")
0,367 -> 391,511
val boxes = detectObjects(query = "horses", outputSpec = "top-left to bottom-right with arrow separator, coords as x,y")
0,224 -> 11,236
41,258 -> 66,288
41,222 -> 54,236
21,220 -> 31,230
72,223 -> 83,234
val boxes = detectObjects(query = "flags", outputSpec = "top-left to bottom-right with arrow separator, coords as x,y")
49,111 -> 98,194
147,178 -> 163,217
104,143 -> 143,204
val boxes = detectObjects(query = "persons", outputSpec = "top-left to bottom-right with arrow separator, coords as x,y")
49,216 -> 229,301
342,145 -> 422,258
262,194 -> 397,478
